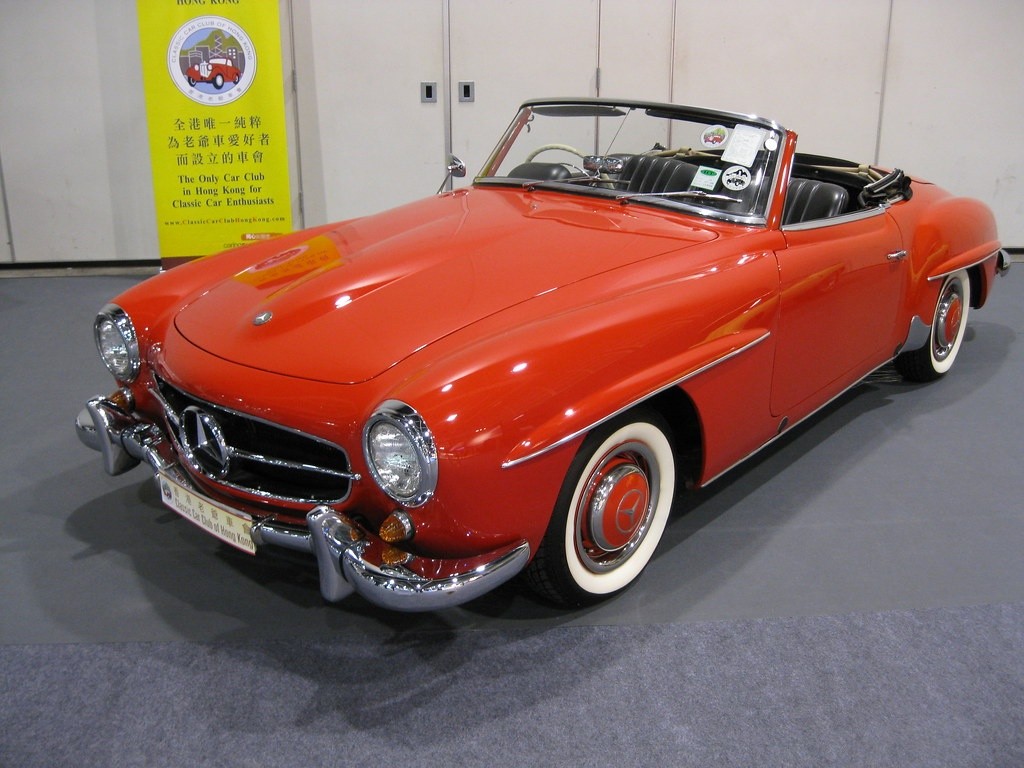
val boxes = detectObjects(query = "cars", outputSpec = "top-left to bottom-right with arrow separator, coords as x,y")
73,96 -> 1012,612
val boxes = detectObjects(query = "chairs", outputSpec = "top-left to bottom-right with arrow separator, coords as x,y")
783,178 -> 848,225
615,155 -> 723,201
